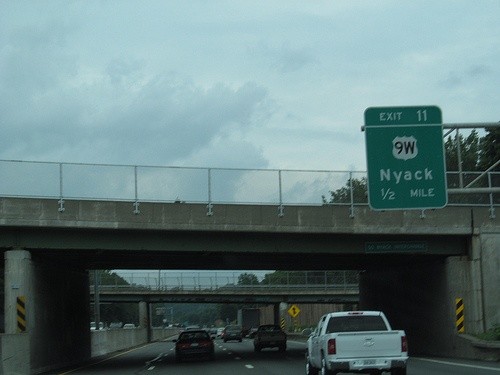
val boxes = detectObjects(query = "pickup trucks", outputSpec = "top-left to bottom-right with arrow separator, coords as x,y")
301,311 -> 410,375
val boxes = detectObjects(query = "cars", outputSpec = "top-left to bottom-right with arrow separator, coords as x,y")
90,322 -> 104,330
223,325 -> 243,341
173,330 -> 216,362
249,324 -> 288,353
186,324 -> 225,337
123,324 -> 135,328
